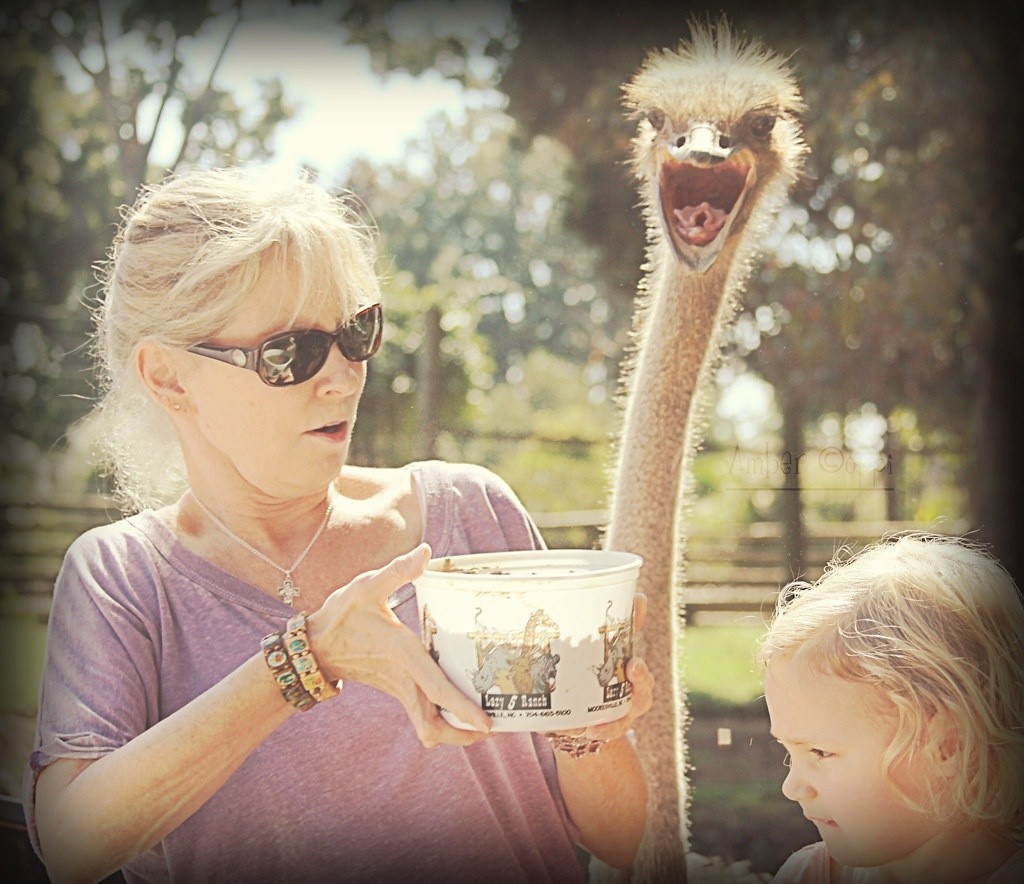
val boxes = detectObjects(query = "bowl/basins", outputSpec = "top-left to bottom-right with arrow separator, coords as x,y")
406,547 -> 643,734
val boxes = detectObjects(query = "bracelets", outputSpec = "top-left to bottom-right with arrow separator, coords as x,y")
262,611 -> 345,713
543,729 -> 611,759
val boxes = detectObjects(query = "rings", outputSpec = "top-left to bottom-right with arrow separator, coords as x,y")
571,727 -> 589,740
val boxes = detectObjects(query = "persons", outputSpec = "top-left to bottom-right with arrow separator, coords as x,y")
757,533 -> 1024,883
22,160 -> 654,883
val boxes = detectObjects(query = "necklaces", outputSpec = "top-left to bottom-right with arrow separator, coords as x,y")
187,488 -> 335,607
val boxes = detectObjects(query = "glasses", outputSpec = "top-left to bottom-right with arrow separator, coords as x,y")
184,301 -> 383,386
